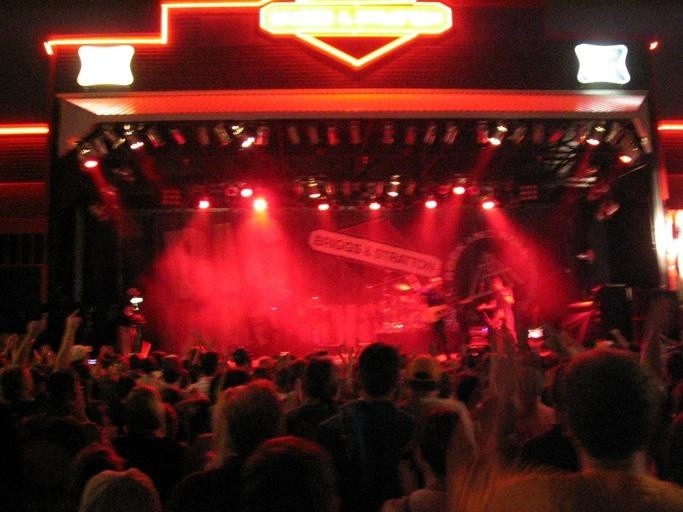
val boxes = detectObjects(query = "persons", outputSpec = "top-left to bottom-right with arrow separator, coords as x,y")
0,308 -> 683,512
118,287 -> 146,355
422,277 -> 452,361
478,276 -> 522,353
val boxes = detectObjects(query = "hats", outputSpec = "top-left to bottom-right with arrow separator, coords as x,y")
406,355 -> 440,383
